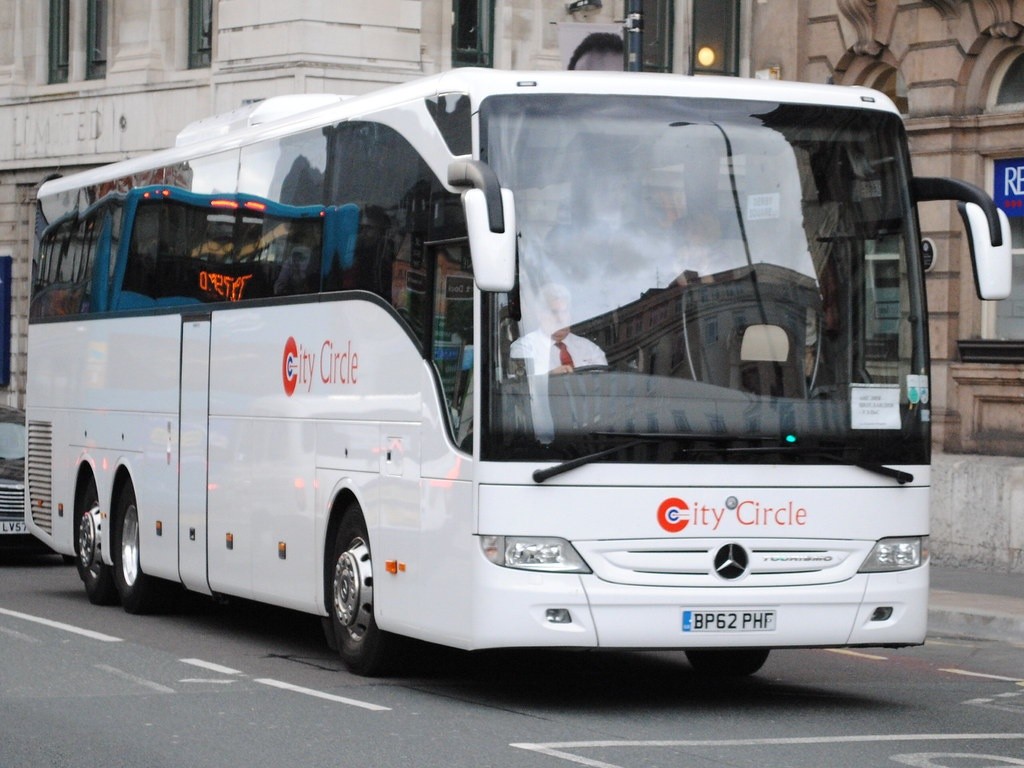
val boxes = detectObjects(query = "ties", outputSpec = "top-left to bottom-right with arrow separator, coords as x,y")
554,341 -> 576,368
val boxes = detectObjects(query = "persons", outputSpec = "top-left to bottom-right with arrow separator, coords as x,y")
506,283 -> 609,378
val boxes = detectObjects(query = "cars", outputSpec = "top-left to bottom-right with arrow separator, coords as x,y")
1,405 -> 31,534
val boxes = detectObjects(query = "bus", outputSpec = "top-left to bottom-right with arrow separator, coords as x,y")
22,66 -> 1015,678
